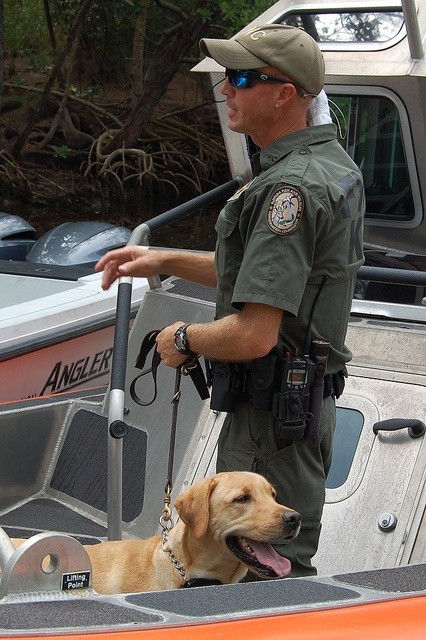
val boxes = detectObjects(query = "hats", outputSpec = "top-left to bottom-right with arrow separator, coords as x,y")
199,24 -> 325,96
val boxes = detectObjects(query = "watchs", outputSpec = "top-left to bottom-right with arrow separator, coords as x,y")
172,324 -> 199,361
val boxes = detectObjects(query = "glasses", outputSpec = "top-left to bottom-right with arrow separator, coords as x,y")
226,68 -> 306,98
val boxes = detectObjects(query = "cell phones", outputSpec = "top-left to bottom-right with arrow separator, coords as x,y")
283,356 -> 313,435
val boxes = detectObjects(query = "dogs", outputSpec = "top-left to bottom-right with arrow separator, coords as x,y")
9,471 -> 302,596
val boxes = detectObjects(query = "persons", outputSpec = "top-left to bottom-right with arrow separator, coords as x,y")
93,23 -> 367,577
